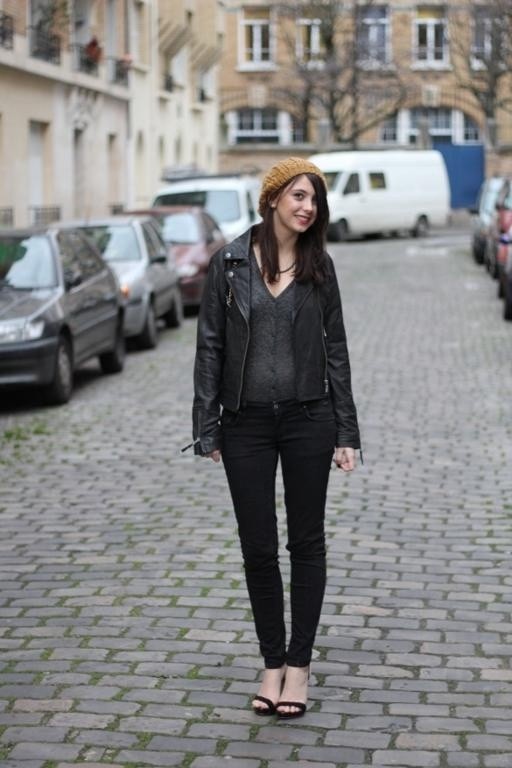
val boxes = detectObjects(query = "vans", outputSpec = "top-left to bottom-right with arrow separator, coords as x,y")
307,149 -> 451,242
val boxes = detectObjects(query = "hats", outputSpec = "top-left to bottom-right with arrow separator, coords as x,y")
257,154 -> 330,218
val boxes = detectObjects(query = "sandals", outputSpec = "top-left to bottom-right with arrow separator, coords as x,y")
249,661 -> 289,717
275,658 -> 312,718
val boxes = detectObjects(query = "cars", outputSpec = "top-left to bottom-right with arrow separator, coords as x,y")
473,175 -> 512,320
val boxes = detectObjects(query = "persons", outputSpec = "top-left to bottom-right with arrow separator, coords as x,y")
187,153 -> 367,719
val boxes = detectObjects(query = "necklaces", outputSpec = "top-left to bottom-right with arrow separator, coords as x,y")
276,259 -> 296,273
